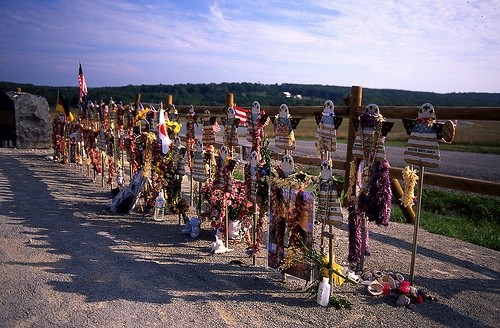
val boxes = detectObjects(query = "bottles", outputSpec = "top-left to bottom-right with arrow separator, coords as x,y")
154,192 -> 166,221
317,277 -> 330,306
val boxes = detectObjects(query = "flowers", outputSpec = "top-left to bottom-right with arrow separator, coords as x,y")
278,233 -> 358,317
201,176 -> 254,226
